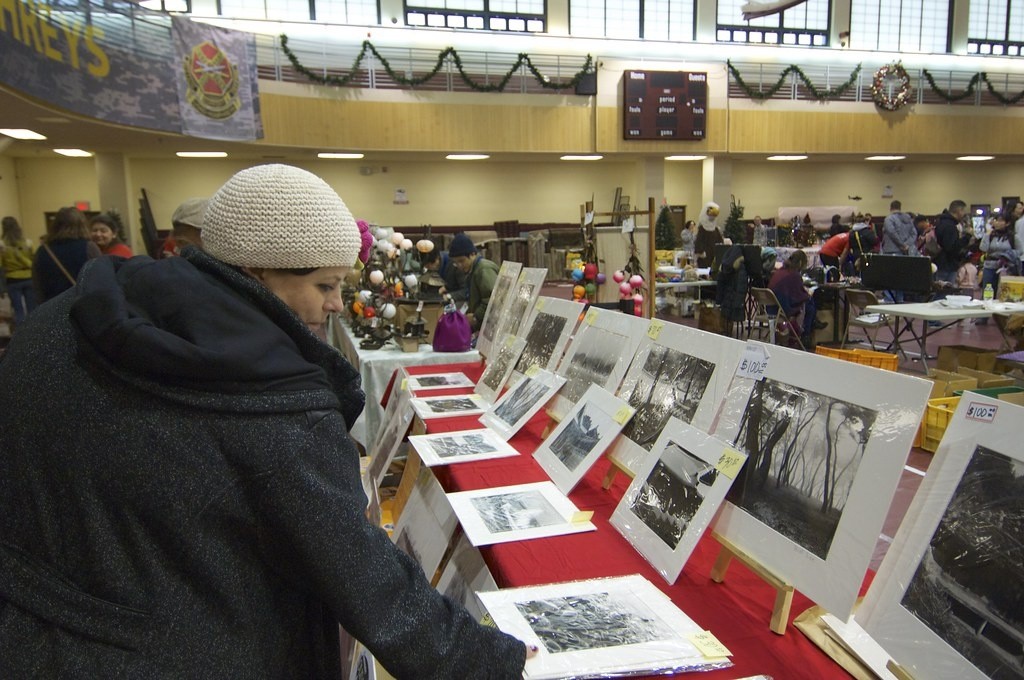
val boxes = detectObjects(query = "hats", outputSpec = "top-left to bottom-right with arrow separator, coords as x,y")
172,197 -> 209,229
448,233 -> 478,258
200,163 -> 373,269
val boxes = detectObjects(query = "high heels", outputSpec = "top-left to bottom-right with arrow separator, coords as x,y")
811,318 -> 828,330
801,337 -> 818,352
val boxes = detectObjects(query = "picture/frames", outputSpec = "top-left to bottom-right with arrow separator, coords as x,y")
609,415 -> 751,587
853,388 -> 1024,680
407,259 -> 750,680
712,340 -> 933,624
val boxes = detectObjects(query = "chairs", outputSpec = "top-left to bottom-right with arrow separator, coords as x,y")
748,287 -> 807,352
841,287 -> 908,360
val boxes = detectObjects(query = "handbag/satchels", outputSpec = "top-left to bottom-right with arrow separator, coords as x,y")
854,257 -> 861,272
433,298 -> 472,352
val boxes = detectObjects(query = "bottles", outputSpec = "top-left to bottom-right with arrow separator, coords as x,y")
984,284 -> 993,308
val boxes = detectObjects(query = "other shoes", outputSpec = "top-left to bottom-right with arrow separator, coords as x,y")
975,318 -> 987,326
928,321 -> 944,328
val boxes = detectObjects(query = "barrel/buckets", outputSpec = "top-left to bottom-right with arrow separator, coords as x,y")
999,281 -> 1024,302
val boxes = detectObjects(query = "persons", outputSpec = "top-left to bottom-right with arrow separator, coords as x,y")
90,215 -> 132,258
680,220 -> 696,251
748,216 -> 767,246
832,200 -> 1024,329
0,164 -> 536,680
819,232 -> 851,280
420,249 -> 467,304
170,198 -> 212,250
766,249 -> 828,352
30,206 -> 103,310
449,235 -> 500,332
0,216 -> 35,323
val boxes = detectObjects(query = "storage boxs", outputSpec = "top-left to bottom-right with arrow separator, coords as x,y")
815,347 -> 899,372
907,345 -> 1023,452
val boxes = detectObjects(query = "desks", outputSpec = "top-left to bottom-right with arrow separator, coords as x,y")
367,360 -> 878,680
656,280 -> 716,329
330,307 -> 483,454
866,302 -> 1024,375
